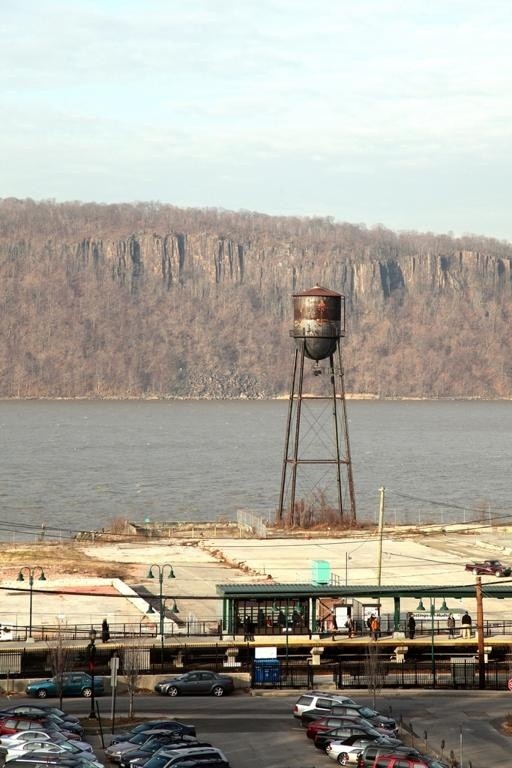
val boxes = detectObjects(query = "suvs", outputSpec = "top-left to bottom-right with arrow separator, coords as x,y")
294,691 -> 360,719
305,716 -> 449,768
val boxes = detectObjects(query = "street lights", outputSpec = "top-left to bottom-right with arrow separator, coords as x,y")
144,562 -> 179,642
16,563 -> 49,643
81,626 -> 98,720
418,589 -> 451,689
145,596 -> 178,670
273,595 -> 304,683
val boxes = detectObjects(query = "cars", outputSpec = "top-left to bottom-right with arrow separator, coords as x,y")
154,670 -> 234,697
25,671 -> 105,699
105,719 -> 230,768
0,704 -> 104,768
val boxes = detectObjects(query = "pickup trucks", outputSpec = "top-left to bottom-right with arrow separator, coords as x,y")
465,558 -> 512,579
300,704 -> 396,731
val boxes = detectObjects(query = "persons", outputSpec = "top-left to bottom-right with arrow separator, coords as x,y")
461,611 -> 472,639
370,617 -> 379,642
345,615 -> 353,639
101,617 -> 112,644
236,608 -> 308,641
408,613 -> 417,640
447,612 -> 456,640
367,614 -> 375,638
